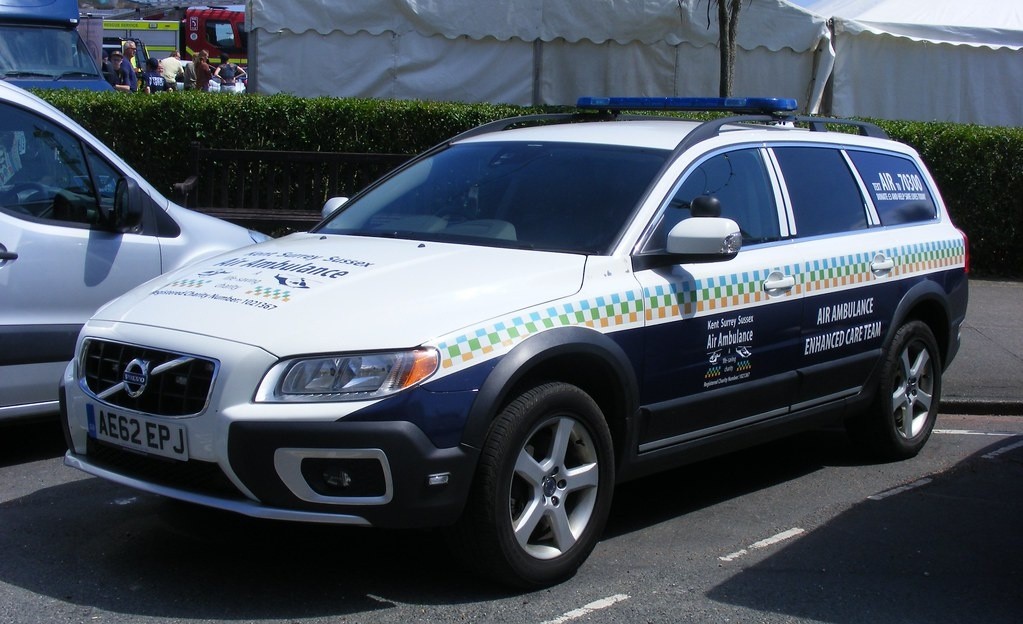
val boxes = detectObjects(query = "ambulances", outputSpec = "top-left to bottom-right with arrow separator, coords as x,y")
59,97 -> 968,590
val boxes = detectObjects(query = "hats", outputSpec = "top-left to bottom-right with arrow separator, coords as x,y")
217,54 -> 229,59
111,51 -> 123,59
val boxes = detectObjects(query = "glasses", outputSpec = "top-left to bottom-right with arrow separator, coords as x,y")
111,58 -> 123,61
128,48 -> 137,50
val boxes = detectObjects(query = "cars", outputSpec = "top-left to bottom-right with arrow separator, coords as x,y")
0,71 -> 277,437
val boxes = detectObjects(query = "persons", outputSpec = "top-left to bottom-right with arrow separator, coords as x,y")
120,41 -> 137,93
109,51 -> 131,91
214,53 -> 246,93
159,51 -> 184,91
195,50 -> 212,91
143,58 -> 173,93
185,54 -> 197,91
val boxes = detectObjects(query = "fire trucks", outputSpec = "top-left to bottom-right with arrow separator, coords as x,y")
102,6 -> 249,84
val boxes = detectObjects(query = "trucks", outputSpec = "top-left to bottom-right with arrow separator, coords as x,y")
0,0 -> 119,95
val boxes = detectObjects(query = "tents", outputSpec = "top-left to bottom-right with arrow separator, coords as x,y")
246,0 -> 1023,126
